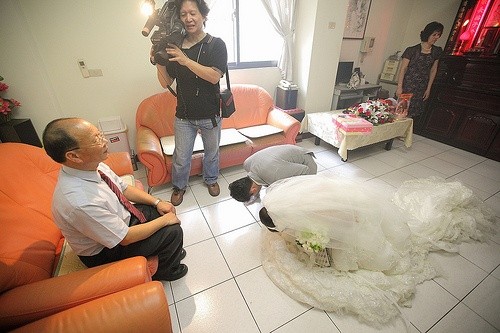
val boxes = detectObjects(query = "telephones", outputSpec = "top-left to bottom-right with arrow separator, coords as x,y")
280,80 -> 297,88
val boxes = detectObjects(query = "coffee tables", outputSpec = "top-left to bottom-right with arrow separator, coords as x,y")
298,106 -> 414,162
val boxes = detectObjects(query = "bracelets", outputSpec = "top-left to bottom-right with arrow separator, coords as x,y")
153,199 -> 160,208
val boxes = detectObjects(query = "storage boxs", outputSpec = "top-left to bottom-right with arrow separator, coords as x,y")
332,115 -> 374,136
379,59 -> 400,81
0,119 -> 44,148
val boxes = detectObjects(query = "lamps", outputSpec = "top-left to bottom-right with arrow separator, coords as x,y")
142,0 -> 156,19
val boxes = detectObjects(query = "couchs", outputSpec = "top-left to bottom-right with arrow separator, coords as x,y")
0,142 -> 174,333
134,84 -> 301,194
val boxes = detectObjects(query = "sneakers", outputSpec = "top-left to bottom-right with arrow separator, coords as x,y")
208,182 -> 220,196
171,185 -> 185,206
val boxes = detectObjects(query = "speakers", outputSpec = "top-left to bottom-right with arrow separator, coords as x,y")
0,118 -> 44,148
276,87 -> 298,110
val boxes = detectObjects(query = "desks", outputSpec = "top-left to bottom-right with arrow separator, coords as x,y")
332,82 -> 383,110
377,75 -> 402,89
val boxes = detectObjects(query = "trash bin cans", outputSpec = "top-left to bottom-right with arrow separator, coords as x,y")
98,116 -> 133,159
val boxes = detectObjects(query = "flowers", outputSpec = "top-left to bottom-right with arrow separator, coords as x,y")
289,226 -> 334,251
350,97 -> 396,125
0,76 -> 22,124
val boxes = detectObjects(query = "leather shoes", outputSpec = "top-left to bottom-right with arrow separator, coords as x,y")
173,248 -> 186,262
152,264 -> 188,281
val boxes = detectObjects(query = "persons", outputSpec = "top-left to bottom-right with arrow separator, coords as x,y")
43,117 -> 187,282
259,174 -> 500,333
150,0 -> 227,206
394,21 -> 444,119
228,144 -> 318,203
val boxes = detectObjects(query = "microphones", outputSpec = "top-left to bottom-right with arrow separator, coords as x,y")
142,10 -> 159,37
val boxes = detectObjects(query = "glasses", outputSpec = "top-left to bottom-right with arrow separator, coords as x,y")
72,130 -> 104,150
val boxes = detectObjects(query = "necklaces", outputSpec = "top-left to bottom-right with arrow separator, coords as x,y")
422,45 -> 432,50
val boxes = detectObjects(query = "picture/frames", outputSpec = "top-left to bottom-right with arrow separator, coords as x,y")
343,0 -> 372,40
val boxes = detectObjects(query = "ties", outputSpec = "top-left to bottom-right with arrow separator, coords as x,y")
98,169 -> 147,223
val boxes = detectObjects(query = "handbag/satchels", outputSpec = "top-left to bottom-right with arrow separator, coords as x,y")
216,89 -> 236,118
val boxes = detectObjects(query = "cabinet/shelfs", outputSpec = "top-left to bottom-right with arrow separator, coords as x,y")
415,88 -> 500,163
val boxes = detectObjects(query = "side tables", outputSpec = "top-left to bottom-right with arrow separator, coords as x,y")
272,103 -> 306,144
